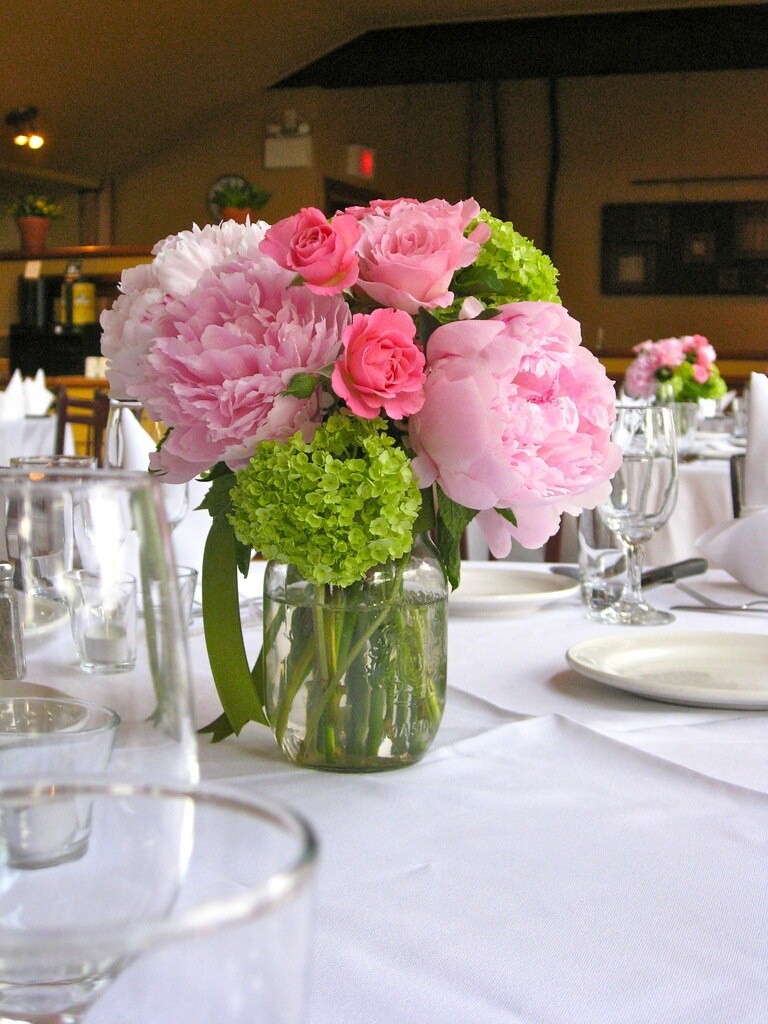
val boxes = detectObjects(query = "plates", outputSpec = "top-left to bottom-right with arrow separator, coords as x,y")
565,632 -> 768,711
401,567 -> 580,617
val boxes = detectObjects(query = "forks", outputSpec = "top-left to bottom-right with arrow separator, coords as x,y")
676,581 -> 768,612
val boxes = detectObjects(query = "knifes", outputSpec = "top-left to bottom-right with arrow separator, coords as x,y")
671,605 -> 768,618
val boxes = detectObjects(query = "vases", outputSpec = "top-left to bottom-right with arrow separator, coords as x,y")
17,215 -> 51,252
263,530 -> 448,772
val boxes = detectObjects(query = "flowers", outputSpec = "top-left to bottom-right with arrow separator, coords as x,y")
7,195 -> 63,219
624,335 -> 729,403
100,198 -> 624,588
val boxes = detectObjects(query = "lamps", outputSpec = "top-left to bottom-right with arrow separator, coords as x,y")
265,124 -> 313,169
7,107 -> 44,149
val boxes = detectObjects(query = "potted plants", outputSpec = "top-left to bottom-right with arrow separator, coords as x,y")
211,180 -> 272,224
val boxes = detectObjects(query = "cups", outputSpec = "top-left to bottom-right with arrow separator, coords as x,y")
579,488 -> 630,615
0,457 -> 317,1024
733,398 -> 748,438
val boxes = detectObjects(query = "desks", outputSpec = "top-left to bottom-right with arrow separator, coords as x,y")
0,415 -> 76,468
0,559 -> 768,1023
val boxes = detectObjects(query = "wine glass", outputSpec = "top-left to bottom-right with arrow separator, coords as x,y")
596,405 -> 676,627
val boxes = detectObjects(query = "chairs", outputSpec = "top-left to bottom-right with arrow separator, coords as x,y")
55,389 -> 109,469
